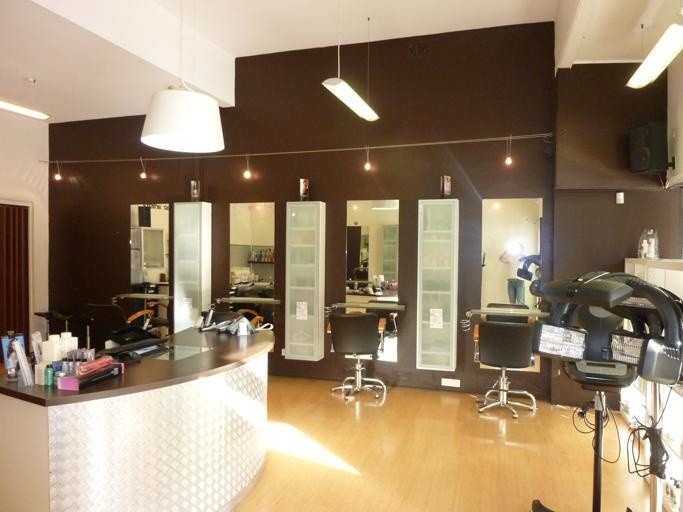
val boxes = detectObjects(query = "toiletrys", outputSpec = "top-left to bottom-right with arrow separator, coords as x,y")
31,329 -> 95,388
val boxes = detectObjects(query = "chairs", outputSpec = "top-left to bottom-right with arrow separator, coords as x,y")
472,321 -> 536,419
325,312 -> 386,402
85,301 -> 160,347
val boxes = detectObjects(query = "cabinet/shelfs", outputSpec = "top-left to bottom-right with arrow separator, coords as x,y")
380,221 -> 398,285
416,198 -> 460,372
284,201 -> 328,363
172,201 -> 213,333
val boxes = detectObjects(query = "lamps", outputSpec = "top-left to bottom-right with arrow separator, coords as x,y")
623,22 -> 683,91
322,0 -> 381,123
139,2 -> 225,154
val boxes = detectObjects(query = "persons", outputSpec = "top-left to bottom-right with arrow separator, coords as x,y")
7,340 -> 24,369
498,241 -> 526,305
6,351 -> 15,369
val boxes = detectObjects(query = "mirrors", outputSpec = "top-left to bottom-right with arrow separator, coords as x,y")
226,201 -> 275,325
343,198 -> 399,314
481,198 -> 544,317
139,225 -> 165,271
128,203 -> 171,320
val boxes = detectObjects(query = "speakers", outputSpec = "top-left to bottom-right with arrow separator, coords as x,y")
628,121 -> 667,175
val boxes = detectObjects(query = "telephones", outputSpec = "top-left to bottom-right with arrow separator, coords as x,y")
200,308 -> 216,332
